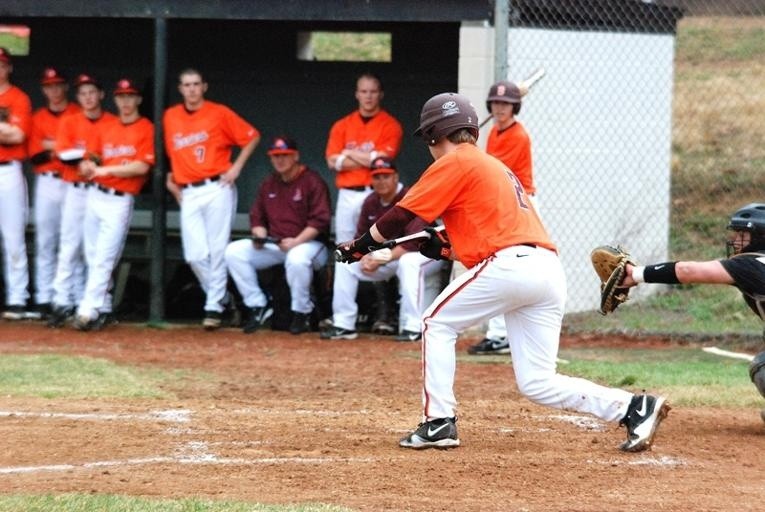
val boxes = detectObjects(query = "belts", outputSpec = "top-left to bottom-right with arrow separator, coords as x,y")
0,161 -> 124,196
344,183 -> 374,191
184,174 -> 220,188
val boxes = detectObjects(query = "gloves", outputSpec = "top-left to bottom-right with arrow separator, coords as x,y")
419,227 -> 451,261
335,229 -> 383,264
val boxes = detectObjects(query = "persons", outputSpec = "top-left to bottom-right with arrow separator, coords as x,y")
70,76 -> 155,330
45,74 -> 110,330
25,69 -> 74,319
162,65 -> 261,330
0,47 -> 32,321
319,156 -> 446,342
467,82 -> 536,355
623,202 -> 765,420
223,136 -> 332,334
317,73 -> 403,332
335,93 -> 673,452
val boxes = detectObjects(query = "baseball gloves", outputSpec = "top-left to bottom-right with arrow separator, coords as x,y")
592,244 -> 636,313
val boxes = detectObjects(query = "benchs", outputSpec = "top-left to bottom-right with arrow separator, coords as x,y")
27,232 -> 398,312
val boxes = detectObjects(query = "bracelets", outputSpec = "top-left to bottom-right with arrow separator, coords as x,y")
335,155 -> 347,171
644,261 -> 682,284
354,229 -> 383,256
632,266 -> 647,284
369,151 -> 377,162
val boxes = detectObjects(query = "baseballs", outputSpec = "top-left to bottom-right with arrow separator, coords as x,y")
371,248 -> 393,264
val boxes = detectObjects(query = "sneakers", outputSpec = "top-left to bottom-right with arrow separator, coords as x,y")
320,326 -> 358,340
290,312 -> 310,333
619,392 -> 669,452
242,300 -> 273,333
465,336 -> 511,354
396,330 -> 423,341
399,416 -> 461,450
204,311 -> 221,328
1,304 -> 119,332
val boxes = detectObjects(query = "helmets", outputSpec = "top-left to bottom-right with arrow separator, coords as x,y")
370,157 -> 397,176
412,92 -> 479,146
266,136 -> 300,155
0,47 -> 141,95
486,81 -> 521,115
726,203 -> 765,258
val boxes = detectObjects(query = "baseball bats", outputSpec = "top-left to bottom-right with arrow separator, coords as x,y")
335,224 -> 446,261
478,66 -> 547,129
